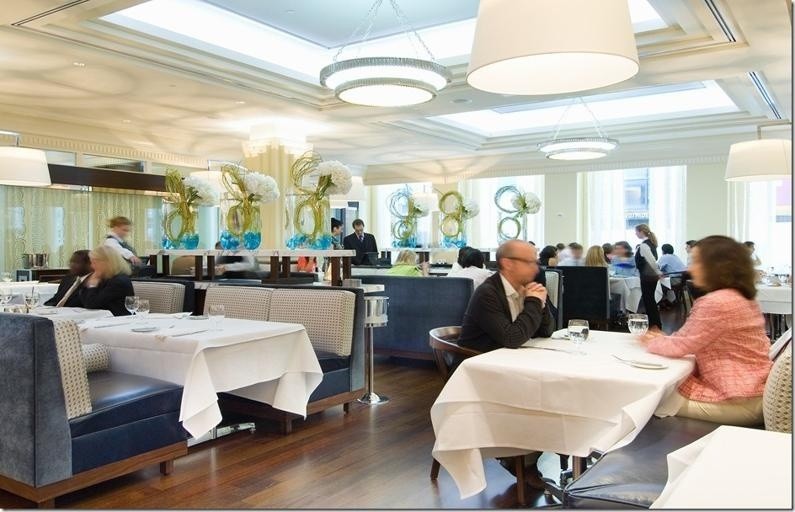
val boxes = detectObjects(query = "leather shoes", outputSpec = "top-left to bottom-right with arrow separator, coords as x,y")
500,457 -> 548,490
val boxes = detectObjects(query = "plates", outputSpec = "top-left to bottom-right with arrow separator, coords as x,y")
630,354 -> 668,370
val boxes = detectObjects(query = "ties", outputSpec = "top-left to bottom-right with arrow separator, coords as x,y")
56,277 -> 81,308
359,235 -> 364,242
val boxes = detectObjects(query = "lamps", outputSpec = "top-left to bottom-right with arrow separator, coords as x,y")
533,94 -> 620,164
465,1 -> 641,93
726,138 -> 792,286
318,0 -> 452,107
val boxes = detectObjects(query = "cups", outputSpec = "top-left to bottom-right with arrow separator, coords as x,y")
36,253 -> 46,266
22,254 -> 33,269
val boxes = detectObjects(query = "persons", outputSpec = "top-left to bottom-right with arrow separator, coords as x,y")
635,235 -> 774,427
450,238 -> 557,491
43,249 -> 93,307
296,216 -> 345,272
386,249 -> 430,277
212,256 -> 256,279
526,240 -> 763,302
65,243 -> 137,317
448,248 -> 497,292
343,219 -> 378,266
635,224 -> 665,331
102,215 -> 143,265
446,245 -> 486,271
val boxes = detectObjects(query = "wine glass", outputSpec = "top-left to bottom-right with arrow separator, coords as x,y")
628,313 -> 649,339
208,303 -> 226,334
124,295 -> 150,320
1,271 -> 14,309
568,319 -> 590,356
24,291 -> 41,315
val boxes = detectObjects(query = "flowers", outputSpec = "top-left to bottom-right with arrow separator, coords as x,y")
493,182 -> 537,244
287,153 -> 349,249
435,184 -> 480,245
158,163 -> 218,247
386,180 -> 441,240
221,164 -> 280,251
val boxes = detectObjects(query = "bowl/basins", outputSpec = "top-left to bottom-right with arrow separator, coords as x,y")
138,256 -> 150,266
